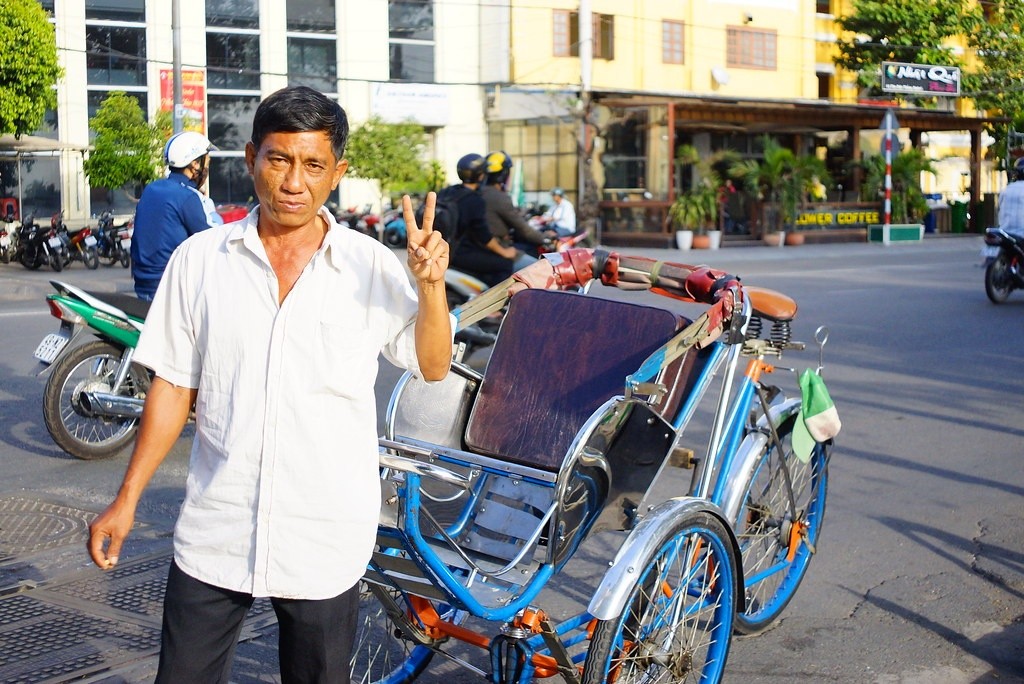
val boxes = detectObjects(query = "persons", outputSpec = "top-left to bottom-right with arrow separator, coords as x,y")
416,151 -> 577,288
130,132 -> 224,303
998,156 -> 1024,252
88,87 -> 458,684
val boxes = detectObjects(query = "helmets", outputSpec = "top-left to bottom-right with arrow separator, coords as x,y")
550,187 -> 563,196
457,153 -> 491,183
1013,157 -> 1024,170
485,150 -> 512,174
165,131 -> 220,168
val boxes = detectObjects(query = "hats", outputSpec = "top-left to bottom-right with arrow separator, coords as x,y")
791,369 -> 842,464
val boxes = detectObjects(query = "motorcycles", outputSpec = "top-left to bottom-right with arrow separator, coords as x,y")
17,208 -> 64,273
94,208 -> 131,268
29,281 -> 198,461
975,227 -> 1024,305
0,210 -> 22,263
51,209 -> 99,270
446,232 -> 588,365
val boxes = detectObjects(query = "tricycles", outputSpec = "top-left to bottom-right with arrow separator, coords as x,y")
348,246 -> 831,684
